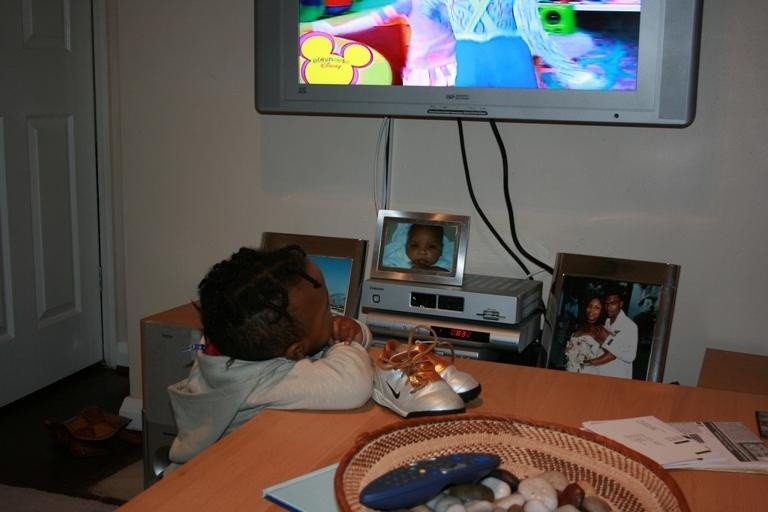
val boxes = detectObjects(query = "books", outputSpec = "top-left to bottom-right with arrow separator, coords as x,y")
579,414 -> 768,474
263,463 -> 340,512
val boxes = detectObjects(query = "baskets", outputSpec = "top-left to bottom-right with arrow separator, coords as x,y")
335,412 -> 690,512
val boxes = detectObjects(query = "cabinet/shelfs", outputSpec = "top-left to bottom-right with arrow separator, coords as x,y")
139,296 -> 207,493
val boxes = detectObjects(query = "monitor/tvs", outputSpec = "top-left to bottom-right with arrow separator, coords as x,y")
253,0 -> 704,129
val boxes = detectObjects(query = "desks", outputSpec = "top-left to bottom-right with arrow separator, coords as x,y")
111,342 -> 766,512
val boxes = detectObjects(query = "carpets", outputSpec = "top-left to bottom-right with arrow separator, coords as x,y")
75,452 -> 146,505
0,482 -> 120,512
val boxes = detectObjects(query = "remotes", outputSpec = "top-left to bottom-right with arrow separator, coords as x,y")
358,452 -> 501,512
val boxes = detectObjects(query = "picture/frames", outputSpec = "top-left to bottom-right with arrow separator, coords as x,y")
258,230 -> 370,326
535,249 -> 682,384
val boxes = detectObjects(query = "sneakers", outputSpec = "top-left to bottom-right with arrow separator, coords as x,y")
371,340 -> 481,417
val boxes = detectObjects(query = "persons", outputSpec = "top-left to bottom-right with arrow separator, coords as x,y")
596,289 -> 639,379
406,225 -> 452,272
162,243 -> 374,479
564,295 -> 615,376
